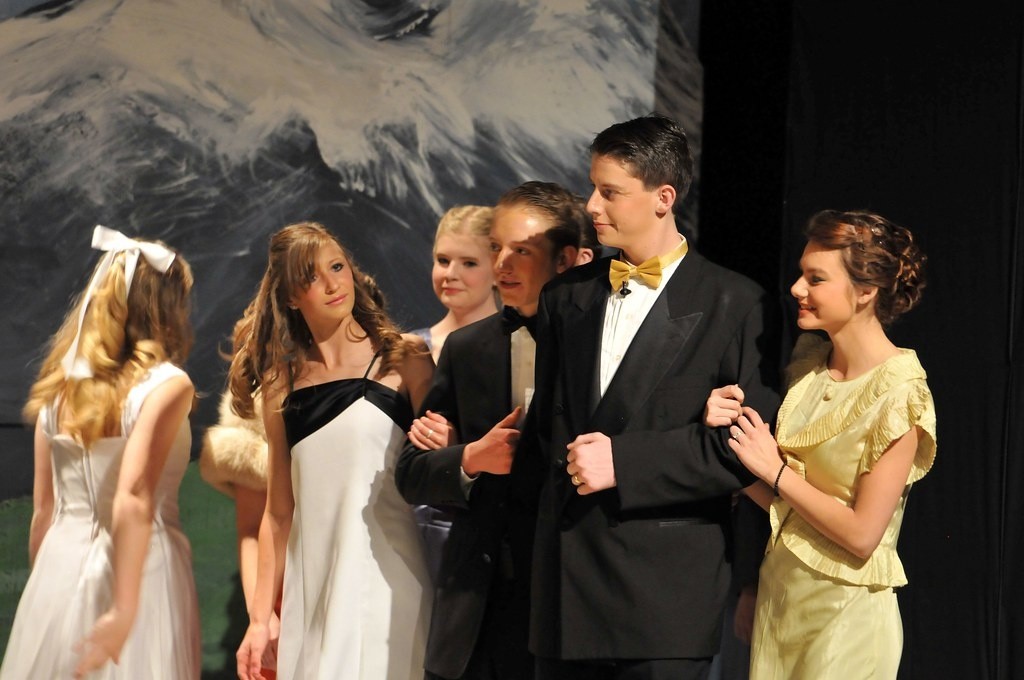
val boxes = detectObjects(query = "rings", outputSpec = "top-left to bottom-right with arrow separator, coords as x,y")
427,429 -> 433,437
733,432 -> 742,438
574,476 -> 580,483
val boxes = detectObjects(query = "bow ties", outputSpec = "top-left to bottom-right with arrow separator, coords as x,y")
609,241 -> 688,292
501,305 -> 538,343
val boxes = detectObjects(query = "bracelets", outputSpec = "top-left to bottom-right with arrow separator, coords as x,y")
775,463 -> 787,496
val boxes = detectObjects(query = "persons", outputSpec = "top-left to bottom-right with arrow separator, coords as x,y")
0,224 -> 203,680
392,182 -> 606,680
704,210 -> 937,679
513,113 -> 786,680
199,222 -> 467,679
